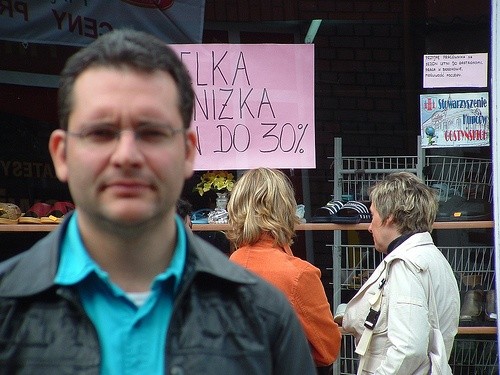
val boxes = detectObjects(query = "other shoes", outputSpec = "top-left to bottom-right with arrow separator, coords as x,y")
484,290 -> 497,327
434,195 -> 467,221
451,199 -> 493,222
333,303 -> 348,326
459,284 -> 484,327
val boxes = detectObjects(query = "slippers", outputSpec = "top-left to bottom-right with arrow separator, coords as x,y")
310,198 -> 342,223
330,200 -> 371,224
0,203 -> 25,225
206,210 -> 228,224
189,208 -> 211,224
41,201 -> 74,224
19,202 -> 53,224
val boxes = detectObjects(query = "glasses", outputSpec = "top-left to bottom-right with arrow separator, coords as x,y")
66,123 -> 186,150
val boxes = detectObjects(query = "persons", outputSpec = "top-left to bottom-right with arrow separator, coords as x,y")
0,29 -> 315,375
340,171 -> 460,375
226,167 -> 341,375
